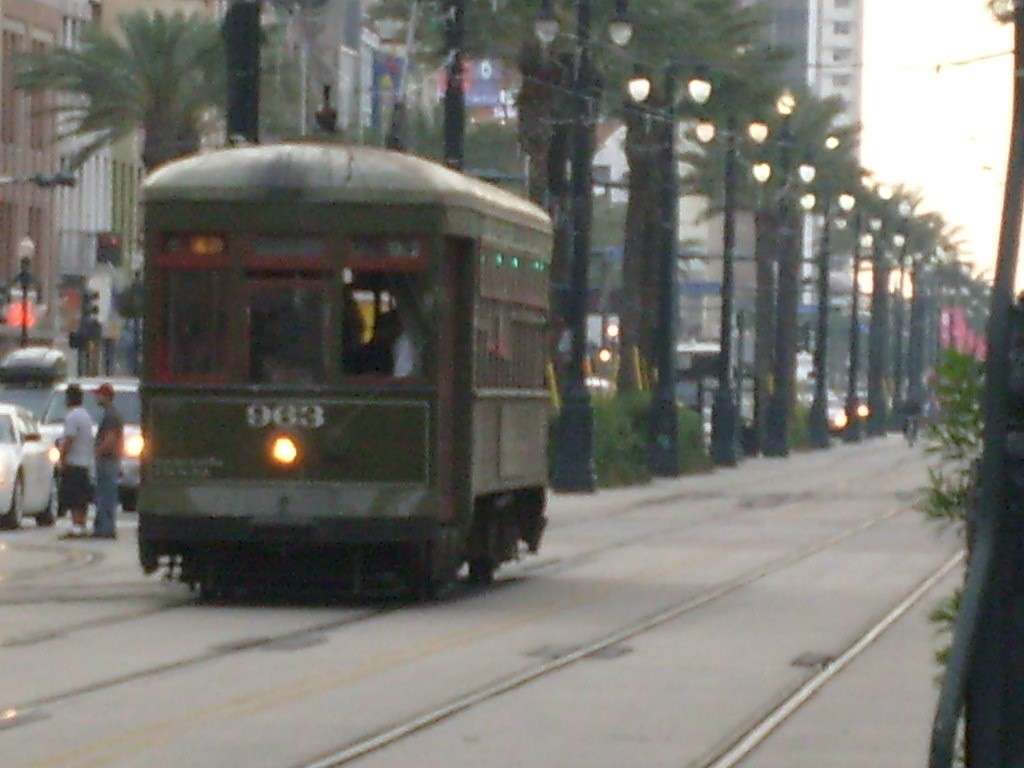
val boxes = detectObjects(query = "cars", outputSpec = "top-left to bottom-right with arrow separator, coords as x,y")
0,402 -> 62,530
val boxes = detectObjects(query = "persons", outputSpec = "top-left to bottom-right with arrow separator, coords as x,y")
343,286 -> 414,377
901,397 -> 920,437
60,383 -> 124,540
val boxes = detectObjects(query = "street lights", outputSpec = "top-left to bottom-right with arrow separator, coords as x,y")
799,132 -> 859,449
620,51 -> 714,476
14,235 -> 39,342
748,92 -> 819,463
695,102 -> 772,466
533,1 -> 636,494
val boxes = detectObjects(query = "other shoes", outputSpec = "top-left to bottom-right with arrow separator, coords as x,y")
89,531 -> 115,539
57,528 -> 81,540
81,527 -> 90,535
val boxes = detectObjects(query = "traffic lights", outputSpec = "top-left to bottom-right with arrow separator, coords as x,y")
80,291 -> 100,332
0,280 -> 10,325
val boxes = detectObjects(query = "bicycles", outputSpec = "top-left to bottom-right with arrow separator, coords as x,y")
900,411 -> 921,447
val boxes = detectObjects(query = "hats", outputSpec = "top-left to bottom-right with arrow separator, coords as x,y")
66,384 -> 83,395
89,382 -> 115,398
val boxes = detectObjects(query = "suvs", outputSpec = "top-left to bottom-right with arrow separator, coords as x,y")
0,342 -> 146,513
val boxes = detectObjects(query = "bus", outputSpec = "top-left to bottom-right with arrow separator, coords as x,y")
109,0 -> 558,605
